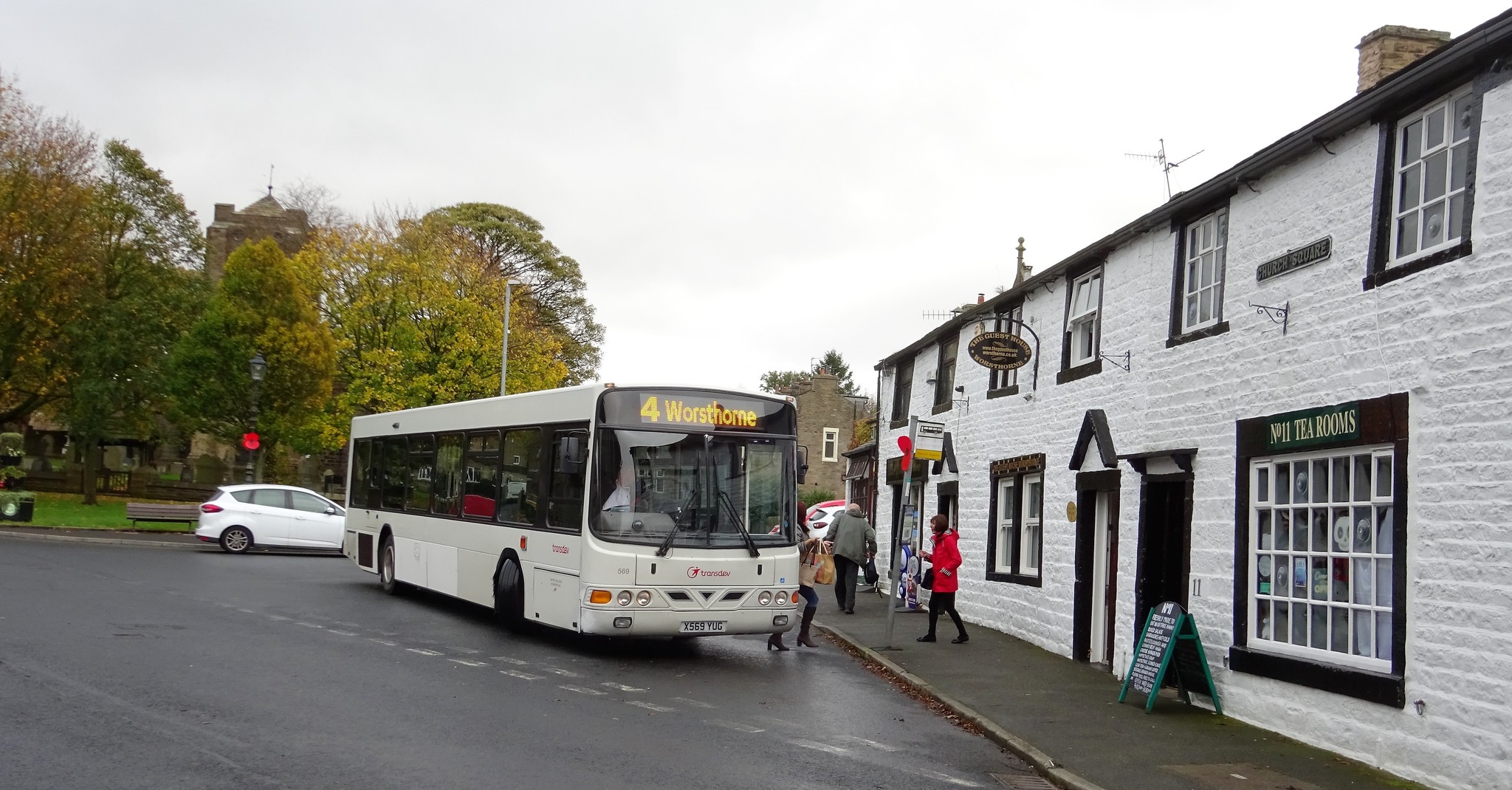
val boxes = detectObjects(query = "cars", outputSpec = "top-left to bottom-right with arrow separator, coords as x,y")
806,505 -> 846,541
770,499 -> 845,534
194,483 -> 346,554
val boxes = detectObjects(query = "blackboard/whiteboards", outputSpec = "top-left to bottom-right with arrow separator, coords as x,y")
1118,601 -> 1223,715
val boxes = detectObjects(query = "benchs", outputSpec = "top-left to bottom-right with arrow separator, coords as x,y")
126,502 -> 200,533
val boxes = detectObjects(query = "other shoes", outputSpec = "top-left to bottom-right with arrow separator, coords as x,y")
951,634 -> 969,644
839,606 -> 845,610
845,609 -> 854,614
917,634 -> 936,642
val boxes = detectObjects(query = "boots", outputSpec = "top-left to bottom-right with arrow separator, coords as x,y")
768,633 -> 789,651
798,607 -> 819,647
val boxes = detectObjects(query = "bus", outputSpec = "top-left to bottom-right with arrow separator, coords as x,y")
338,382 -> 811,649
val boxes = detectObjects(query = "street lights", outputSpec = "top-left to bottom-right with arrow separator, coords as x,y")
500,279 -> 521,396
244,348 -> 268,483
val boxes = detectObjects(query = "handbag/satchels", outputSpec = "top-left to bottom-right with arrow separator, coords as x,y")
799,539 -> 819,588
864,557 -> 879,590
808,539 -> 834,584
920,568 -> 934,590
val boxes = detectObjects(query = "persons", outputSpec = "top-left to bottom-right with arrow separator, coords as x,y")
824,503 -> 877,614
767,501 -> 819,652
600,467 -> 648,513
916,515 -> 969,644
